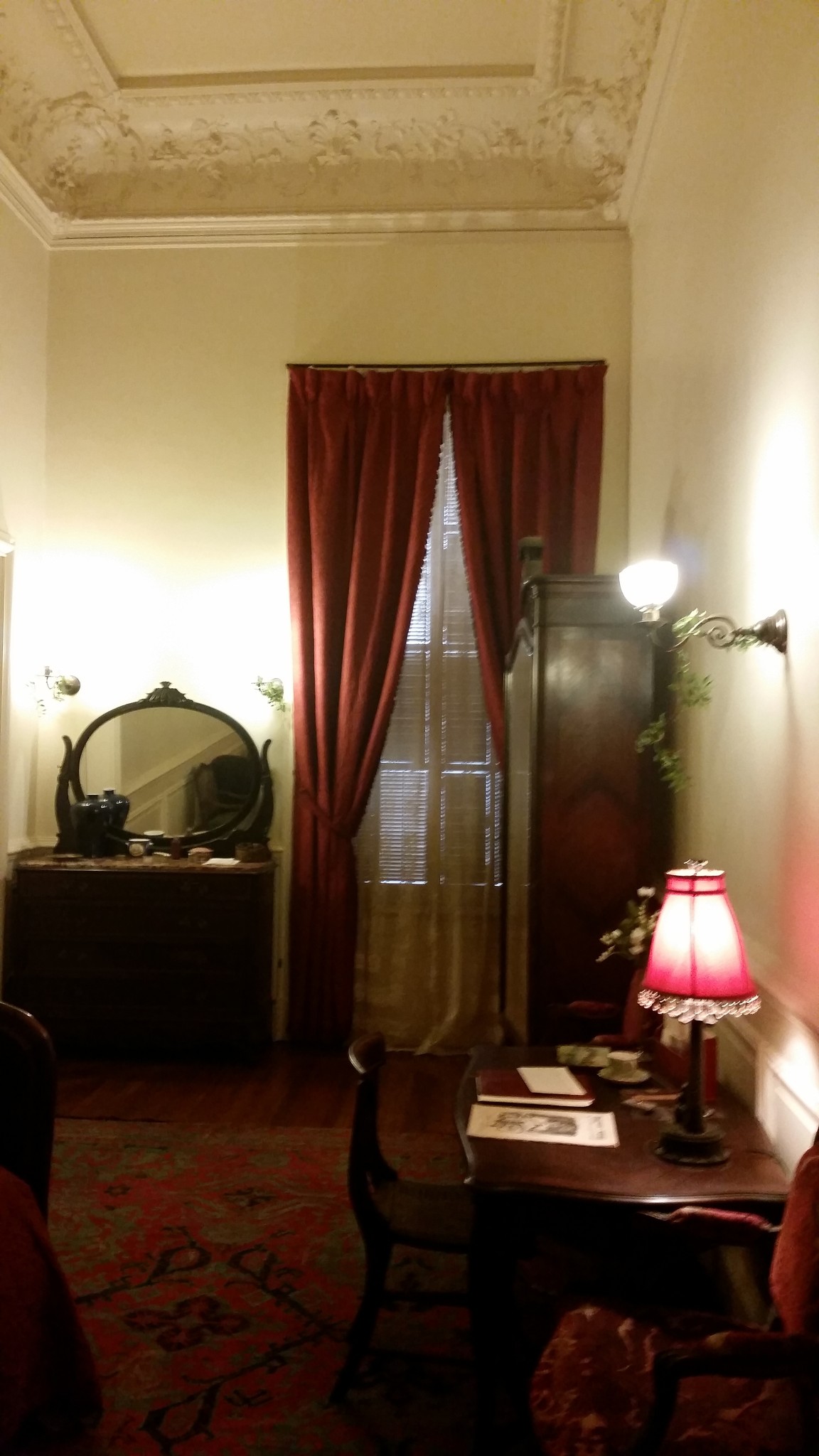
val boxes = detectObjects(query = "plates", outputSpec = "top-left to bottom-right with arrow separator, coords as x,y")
556,1044 -> 612,1068
49,854 -> 84,861
598,1067 -> 652,1084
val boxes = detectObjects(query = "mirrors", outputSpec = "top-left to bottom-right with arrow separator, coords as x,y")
46,680 -> 274,855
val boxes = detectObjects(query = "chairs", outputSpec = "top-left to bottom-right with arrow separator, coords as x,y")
0,1000 -> 57,1207
529,1132 -> 819,1456
324,1027 -> 481,1407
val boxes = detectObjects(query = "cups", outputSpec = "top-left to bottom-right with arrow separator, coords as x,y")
144,830 -> 164,839
606,1051 -> 638,1078
125,839 -> 154,858
188,846 -> 214,863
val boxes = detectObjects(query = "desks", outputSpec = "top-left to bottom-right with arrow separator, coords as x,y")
0,1163 -> 104,1456
450,1039 -> 794,1456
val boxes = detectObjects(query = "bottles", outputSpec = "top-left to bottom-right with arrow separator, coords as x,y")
98,787 -> 129,831
68,793 -> 111,857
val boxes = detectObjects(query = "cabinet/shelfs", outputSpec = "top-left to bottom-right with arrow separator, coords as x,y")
3,863 -> 275,1061
497,534 -> 666,1052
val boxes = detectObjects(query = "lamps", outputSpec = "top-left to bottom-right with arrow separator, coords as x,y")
632,857 -> 769,1171
615,548 -> 795,666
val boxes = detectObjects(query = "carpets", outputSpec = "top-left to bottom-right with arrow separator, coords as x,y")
46,1112 -> 593,1456
